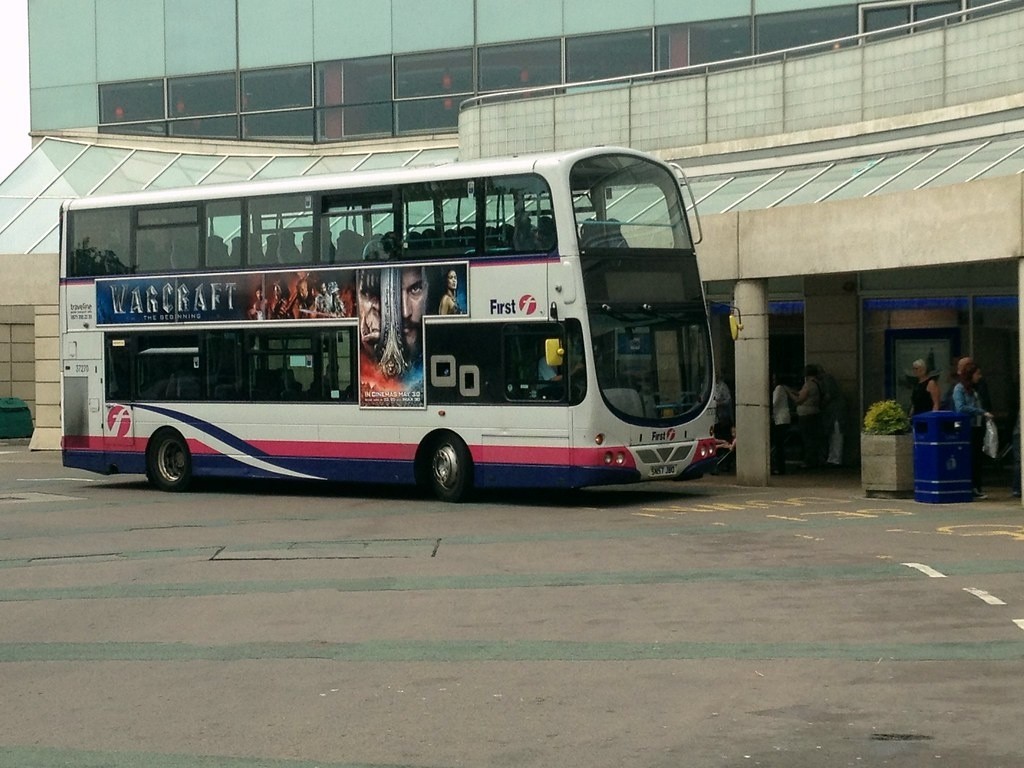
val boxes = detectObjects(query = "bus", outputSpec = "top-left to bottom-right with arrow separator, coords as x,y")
58,145 -> 745,504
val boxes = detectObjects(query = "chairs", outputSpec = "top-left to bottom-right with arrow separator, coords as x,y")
71,215 -> 629,277
522,360 -> 546,394
284,369 -> 302,399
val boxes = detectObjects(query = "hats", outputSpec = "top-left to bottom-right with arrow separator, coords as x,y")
957,357 -> 975,375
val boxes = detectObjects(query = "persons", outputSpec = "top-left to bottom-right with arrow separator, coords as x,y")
907,359 -> 940,420
715,371 -> 736,456
402,266 -> 461,354
538,355 -> 562,382
256,271 -> 346,319
953,357 -> 994,500
770,364 -> 826,477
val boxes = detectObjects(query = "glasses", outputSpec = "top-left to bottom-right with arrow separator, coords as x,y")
912,365 -> 918,370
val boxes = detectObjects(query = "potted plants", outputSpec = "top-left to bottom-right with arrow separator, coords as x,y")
860,399 -> 914,499
